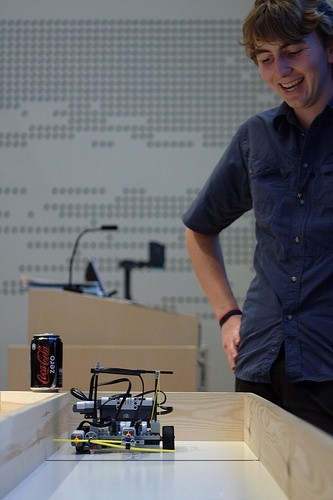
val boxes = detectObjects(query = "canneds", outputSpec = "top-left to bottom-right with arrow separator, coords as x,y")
28,333 -> 63,392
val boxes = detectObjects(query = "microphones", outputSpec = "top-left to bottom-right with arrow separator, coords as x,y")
68,225 -> 118,284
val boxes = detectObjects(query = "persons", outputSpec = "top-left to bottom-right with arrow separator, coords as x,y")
182,0 -> 333,439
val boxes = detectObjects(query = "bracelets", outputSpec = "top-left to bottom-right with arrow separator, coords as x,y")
219,310 -> 241,326
